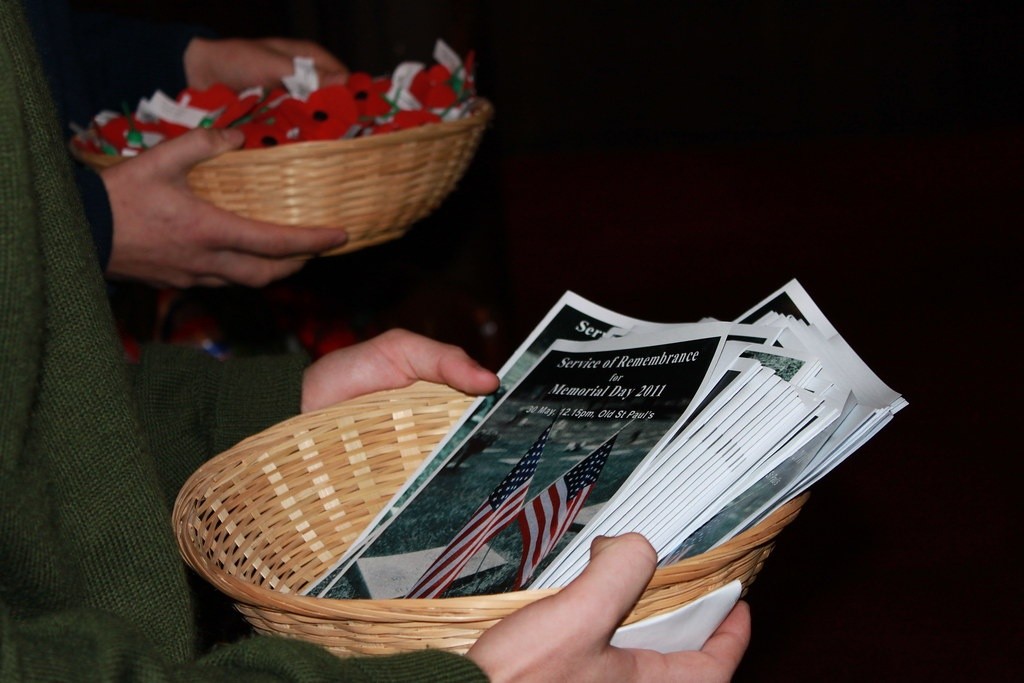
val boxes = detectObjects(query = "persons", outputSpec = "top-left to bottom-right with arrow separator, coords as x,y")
1,0 -> 753,683
70,39 -> 356,291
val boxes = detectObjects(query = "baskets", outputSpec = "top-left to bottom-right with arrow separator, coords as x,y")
69,96 -> 495,262
169,376 -> 809,663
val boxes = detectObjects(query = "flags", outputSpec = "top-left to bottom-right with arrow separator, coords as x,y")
403,422 -> 556,599
511,433 -> 620,592
